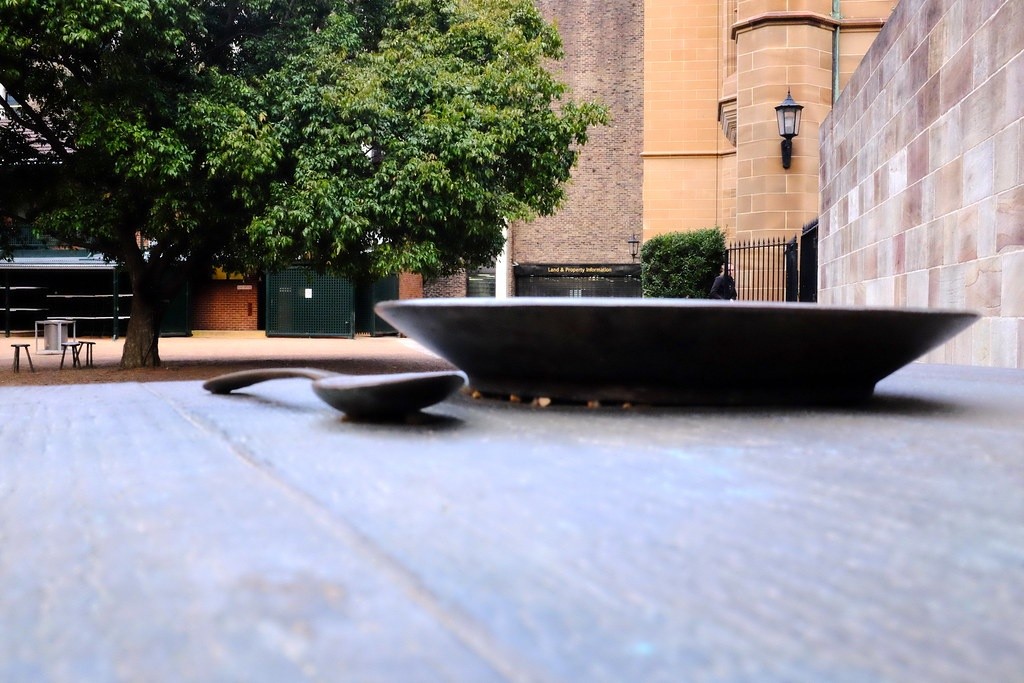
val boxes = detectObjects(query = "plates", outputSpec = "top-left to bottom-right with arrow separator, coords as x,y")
374,293 -> 984,404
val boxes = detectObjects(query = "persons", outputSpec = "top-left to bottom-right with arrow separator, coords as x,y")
710,263 -> 738,302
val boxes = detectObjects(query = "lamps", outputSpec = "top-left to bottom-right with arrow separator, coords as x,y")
774,86 -> 804,169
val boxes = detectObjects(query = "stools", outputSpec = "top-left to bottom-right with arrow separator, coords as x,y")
73,341 -> 96,367
60,342 -> 82,371
11,344 -> 35,374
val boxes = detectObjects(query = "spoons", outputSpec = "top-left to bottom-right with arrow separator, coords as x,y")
203,365 -> 470,421
